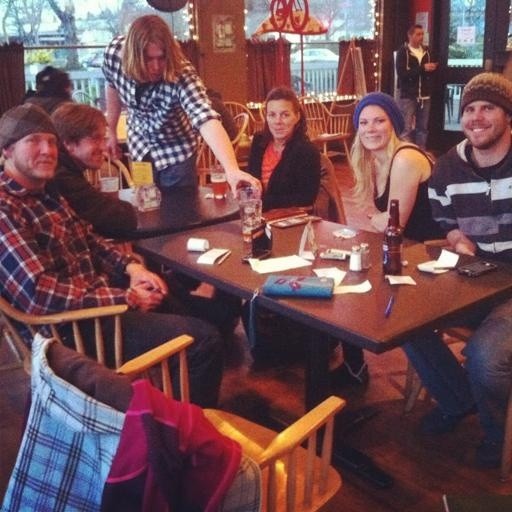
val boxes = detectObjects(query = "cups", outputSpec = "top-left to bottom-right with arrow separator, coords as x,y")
97,175 -> 120,197
236,188 -> 260,205
240,199 -> 262,256
208,164 -> 231,201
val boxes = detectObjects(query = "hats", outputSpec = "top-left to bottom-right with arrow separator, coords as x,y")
353,92 -> 404,137
0,103 -> 59,149
461,73 -> 512,115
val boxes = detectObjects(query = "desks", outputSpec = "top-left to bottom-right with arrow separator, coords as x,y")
106,183 -> 510,487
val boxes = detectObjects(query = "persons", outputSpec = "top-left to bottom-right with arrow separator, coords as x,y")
100,14 -> 263,201
0,102 -> 225,408
19,65 -> 78,115
239,85 -> 321,374
399,72 -> 511,469
397,23 -> 440,151
325,91 -> 450,390
45,102 -> 139,243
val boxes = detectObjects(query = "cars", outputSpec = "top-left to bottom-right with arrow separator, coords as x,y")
290,48 -> 339,71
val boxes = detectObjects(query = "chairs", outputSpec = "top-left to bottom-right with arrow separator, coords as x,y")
1,292 -> 132,373
265,150 -> 334,213
2,95 -> 358,191
25,329 -> 349,511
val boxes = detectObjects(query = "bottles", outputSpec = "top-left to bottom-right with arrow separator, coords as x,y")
347,245 -> 363,271
361,244 -> 373,269
382,198 -> 404,274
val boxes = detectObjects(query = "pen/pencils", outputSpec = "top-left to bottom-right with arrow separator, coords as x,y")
385,296 -> 394,318
218,251 -> 231,265
434,267 -> 458,270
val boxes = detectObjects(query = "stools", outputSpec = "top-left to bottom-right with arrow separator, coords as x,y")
402,327 -> 511,482
